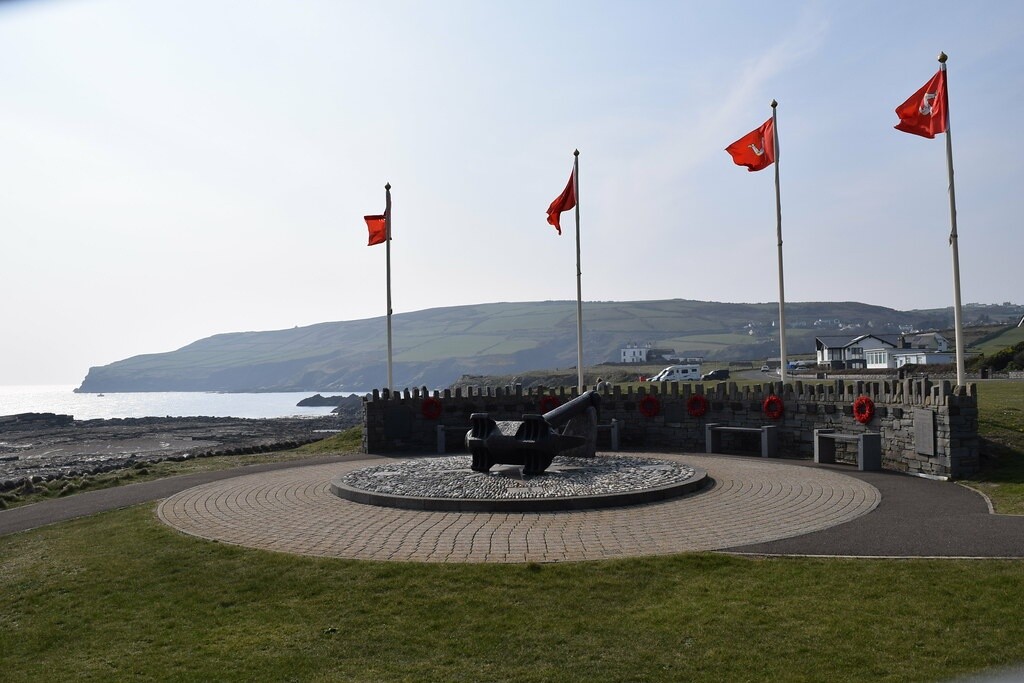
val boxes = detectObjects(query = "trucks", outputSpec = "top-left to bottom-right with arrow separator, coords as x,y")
651,364 -> 701,382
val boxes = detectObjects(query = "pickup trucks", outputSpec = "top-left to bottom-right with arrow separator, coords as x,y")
701,370 -> 730,381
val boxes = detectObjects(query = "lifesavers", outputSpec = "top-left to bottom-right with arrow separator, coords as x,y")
762,395 -> 784,421
540,396 -> 560,414
420,396 -> 443,421
686,394 -> 708,418
852,396 -> 875,425
638,394 -> 662,417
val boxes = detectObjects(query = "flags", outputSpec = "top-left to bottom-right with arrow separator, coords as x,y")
724,117 -> 778,172
893,70 -> 948,139
364,200 -> 392,246
546,161 -> 577,235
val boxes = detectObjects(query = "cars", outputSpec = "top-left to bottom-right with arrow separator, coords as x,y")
795,366 -> 809,370
761,365 -> 769,372
